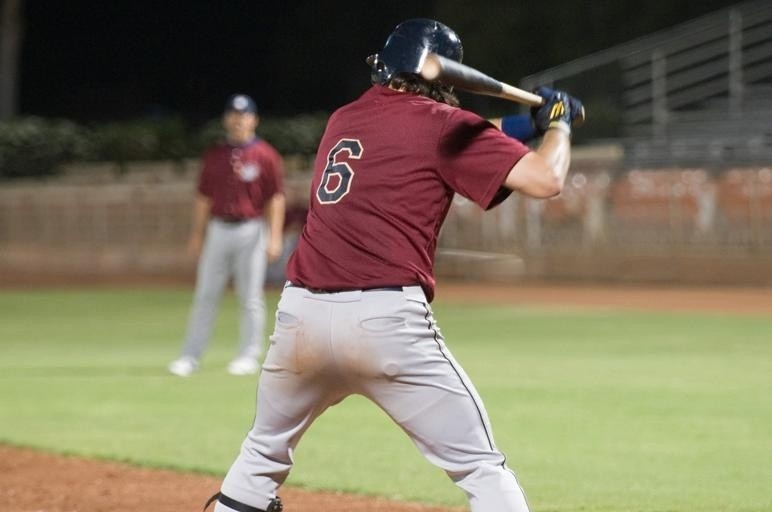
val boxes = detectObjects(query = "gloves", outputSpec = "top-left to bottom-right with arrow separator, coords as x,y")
530,85 -> 586,131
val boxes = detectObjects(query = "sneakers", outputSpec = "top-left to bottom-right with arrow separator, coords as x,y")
227,356 -> 257,375
170,358 -> 195,375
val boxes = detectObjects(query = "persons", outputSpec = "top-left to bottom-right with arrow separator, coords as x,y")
163,92 -> 286,379
202,14 -> 583,512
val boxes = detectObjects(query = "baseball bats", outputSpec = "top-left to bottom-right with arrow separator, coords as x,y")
422,53 -> 586,125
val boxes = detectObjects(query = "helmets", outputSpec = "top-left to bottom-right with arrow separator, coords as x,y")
225,94 -> 257,113
365,18 -> 464,108
